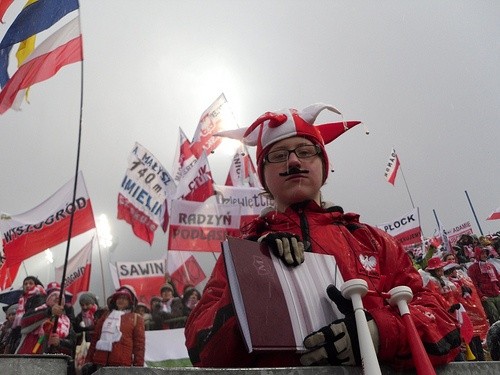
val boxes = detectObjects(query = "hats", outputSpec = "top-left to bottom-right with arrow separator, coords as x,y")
211,104 -> 361,193
6,304 -> 18,317
443,260 -> 461,271
79,293 -> 97,305
161,284 -> 174,296
45,282 -> 65,305
425,256 -> 448,271
112,288 -> 133,306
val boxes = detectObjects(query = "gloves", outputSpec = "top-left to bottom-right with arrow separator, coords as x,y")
300,284 -> 378,366
461,285 -> 472,298
257,233 -> 310,267
448,303 -> 460,313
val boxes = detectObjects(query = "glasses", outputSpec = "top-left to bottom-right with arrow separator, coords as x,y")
263,144 -> 321,165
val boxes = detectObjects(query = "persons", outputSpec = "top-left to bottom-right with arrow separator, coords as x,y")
184,103 -> 461,368
0,276 -> 202,370
406,229 -> 500,363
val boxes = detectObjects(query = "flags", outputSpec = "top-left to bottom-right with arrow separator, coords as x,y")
0,0 -> 82,117
55,238 -> 93,303
167,246 -> 206,296
161,94 -> 260,251
117,141 -> 177,247
376,208 -> 421,246
384,149 -> 400,185
0,172 -> 96,290
